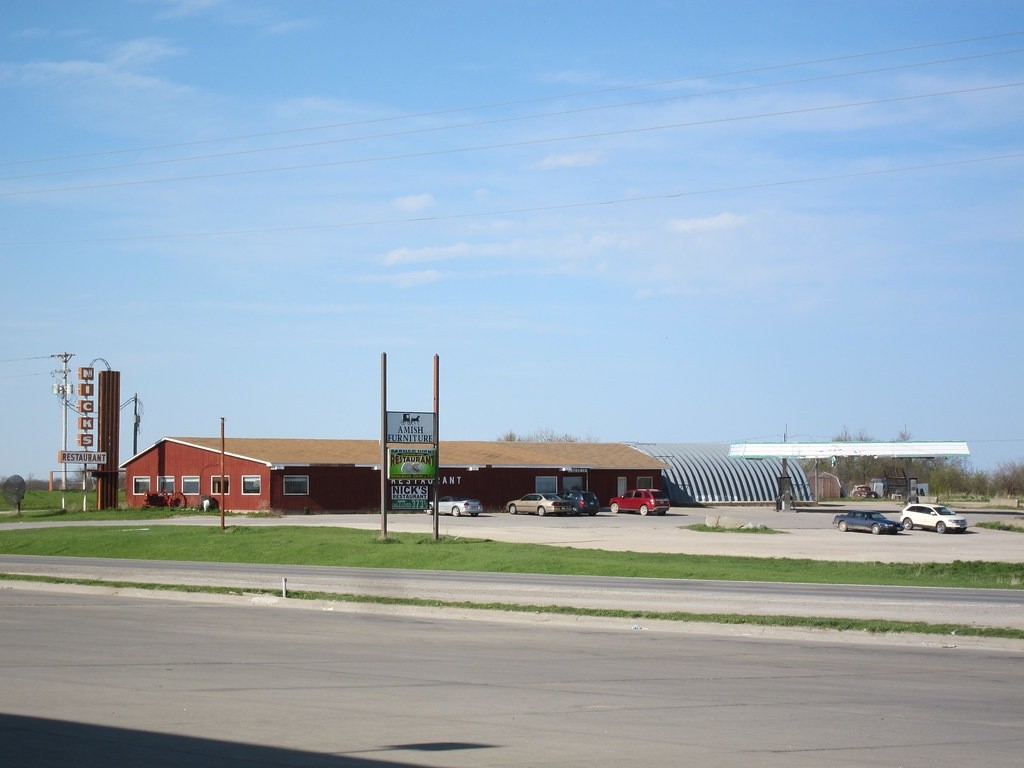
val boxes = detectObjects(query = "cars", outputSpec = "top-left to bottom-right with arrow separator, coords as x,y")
556,491 -> 600,517
900,504 -> 967,534
833,510 -> 905,535
850,485 -> 877,499
609,489 -> 670,516
506,493 -> 572,516
423,495 -> 484,517
